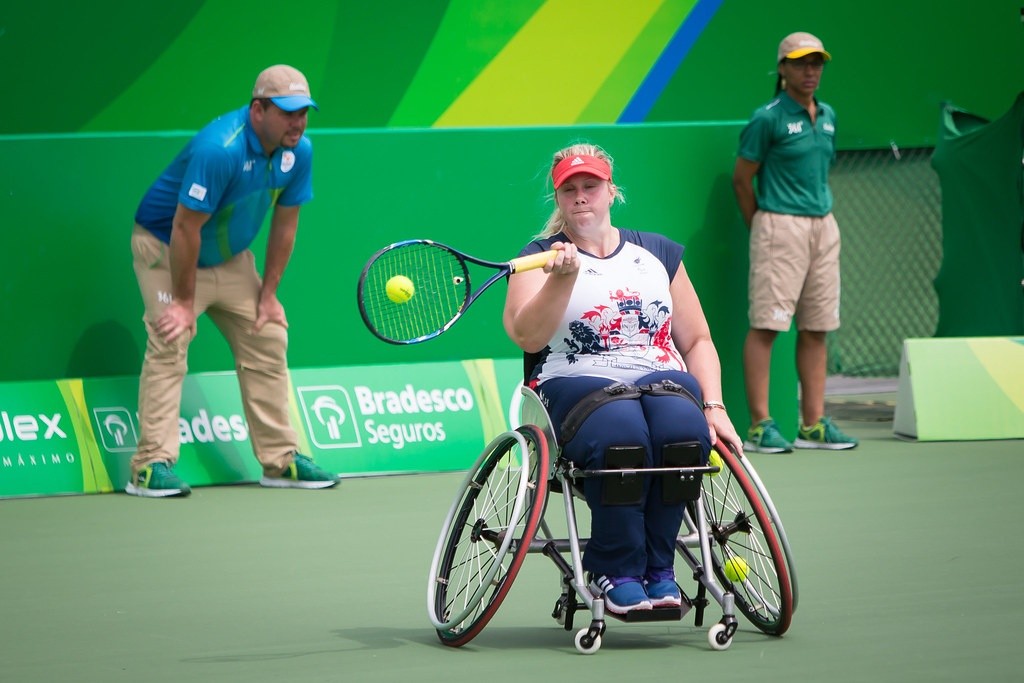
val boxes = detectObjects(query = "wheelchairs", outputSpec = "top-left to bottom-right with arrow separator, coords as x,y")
425,341 -> 800,656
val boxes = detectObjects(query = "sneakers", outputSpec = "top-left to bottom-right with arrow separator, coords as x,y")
794,415 -> 858,450
259,454 -> 341,489
125,462 -> 191,497
743,416 -> 794,454
587,571 -> 681,614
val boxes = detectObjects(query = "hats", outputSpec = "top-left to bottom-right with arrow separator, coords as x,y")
253,64 -> 320,112
778,32 -> 831,64
553,155 -> 612,190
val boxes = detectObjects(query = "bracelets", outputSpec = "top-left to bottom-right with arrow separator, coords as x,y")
703,400 -> 727,411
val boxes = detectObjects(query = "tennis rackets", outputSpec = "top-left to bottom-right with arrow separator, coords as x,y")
356,238 -> 558,347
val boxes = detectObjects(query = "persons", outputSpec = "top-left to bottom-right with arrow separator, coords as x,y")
734,32 -> 859,453
503,144 -> 744,614
124,64 -> 341,496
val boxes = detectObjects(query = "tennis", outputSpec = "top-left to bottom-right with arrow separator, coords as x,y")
725,556 -> 749,582
384,274 -> 416,305
702,449 -> 725,478
